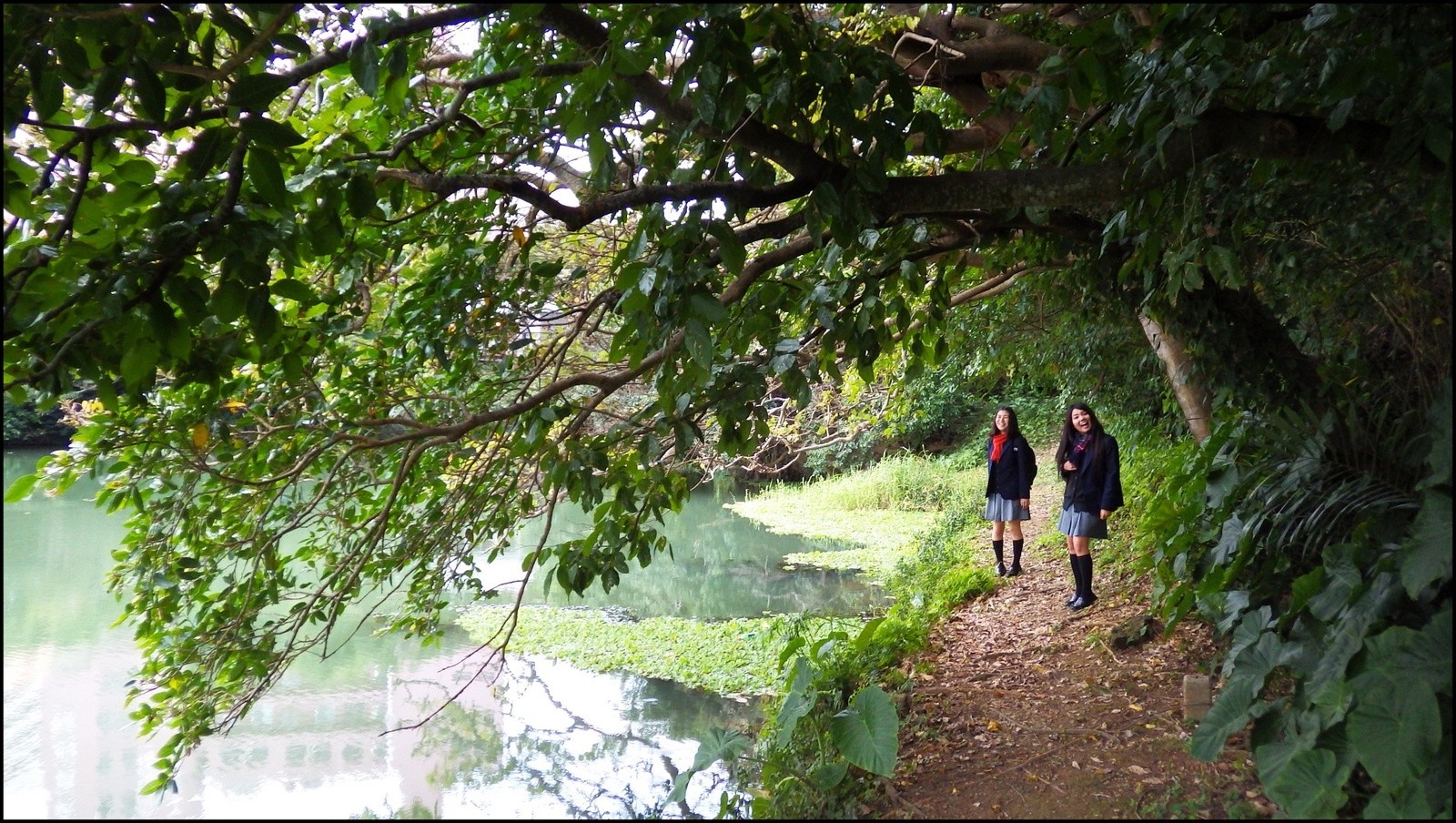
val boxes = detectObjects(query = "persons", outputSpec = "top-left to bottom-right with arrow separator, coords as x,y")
1055,404 -> 1120,613
985,405 -> 1033,577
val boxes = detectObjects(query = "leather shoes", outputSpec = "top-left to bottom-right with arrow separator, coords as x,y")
1009,562 -> 1021,576
1065,593 -> 1078,606
995,561 -> 1006,576
1072,593 -> 1097,609
